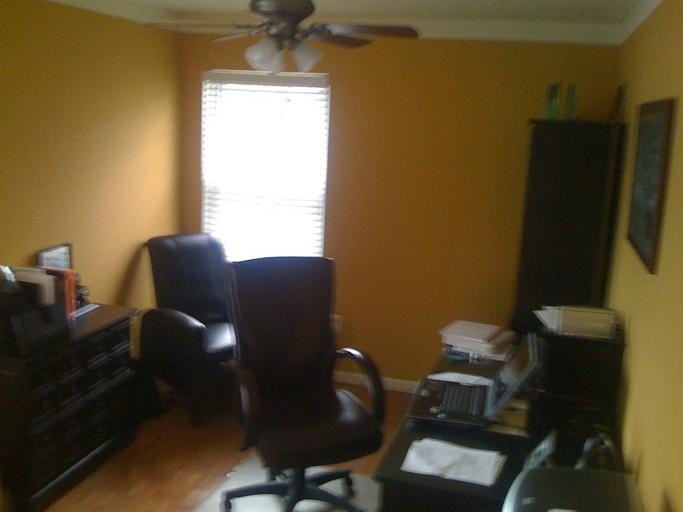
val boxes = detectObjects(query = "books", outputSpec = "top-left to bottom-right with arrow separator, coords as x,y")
437,320 -> 521,362
532,305 -> 620,338
0,265 -> 56,307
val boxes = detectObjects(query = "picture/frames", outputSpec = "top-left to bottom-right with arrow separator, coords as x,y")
38,240 -> 73,280
627,100 -> 671,275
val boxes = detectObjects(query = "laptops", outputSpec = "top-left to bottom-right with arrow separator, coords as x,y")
404,330 -> 539,429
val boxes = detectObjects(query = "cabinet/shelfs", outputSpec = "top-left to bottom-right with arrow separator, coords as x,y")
0,302 -> 140,511
513,118 -> 624,334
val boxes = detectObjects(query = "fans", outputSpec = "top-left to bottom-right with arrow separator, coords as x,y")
171,2 -> 417,49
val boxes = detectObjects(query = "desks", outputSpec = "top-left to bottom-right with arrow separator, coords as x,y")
372,336 -> 628,511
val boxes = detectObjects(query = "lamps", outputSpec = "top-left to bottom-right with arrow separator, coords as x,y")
244,36 -> 327,77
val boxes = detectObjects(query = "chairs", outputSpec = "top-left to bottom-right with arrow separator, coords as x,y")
143,233 -> 236,405
218,258 -> 386,511
